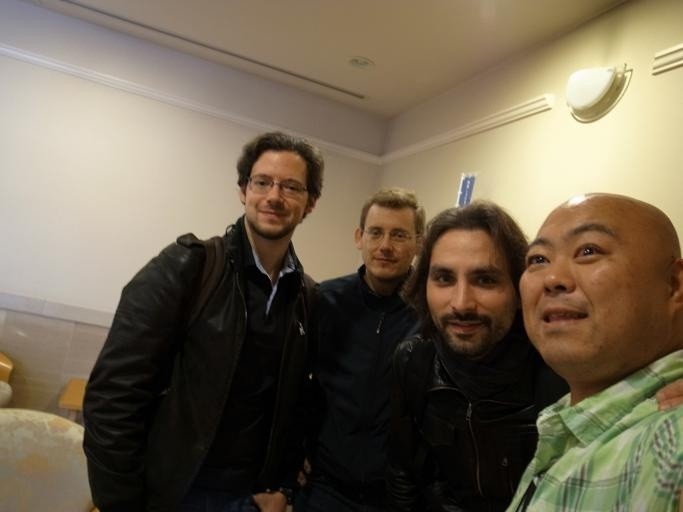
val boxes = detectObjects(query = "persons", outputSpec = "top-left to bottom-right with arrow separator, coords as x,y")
502,191 -> 681,511
314,185 -> 423,512
80,130 -> 312,511
305,200 -> 680,511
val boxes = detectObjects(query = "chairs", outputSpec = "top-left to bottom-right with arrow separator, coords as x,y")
0,408 -> 95,511
0,352 -> 14,407
58,375 -> 87,421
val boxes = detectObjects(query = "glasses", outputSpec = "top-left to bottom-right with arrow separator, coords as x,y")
363,225 -> 422,241
246,174 -> 310,196
